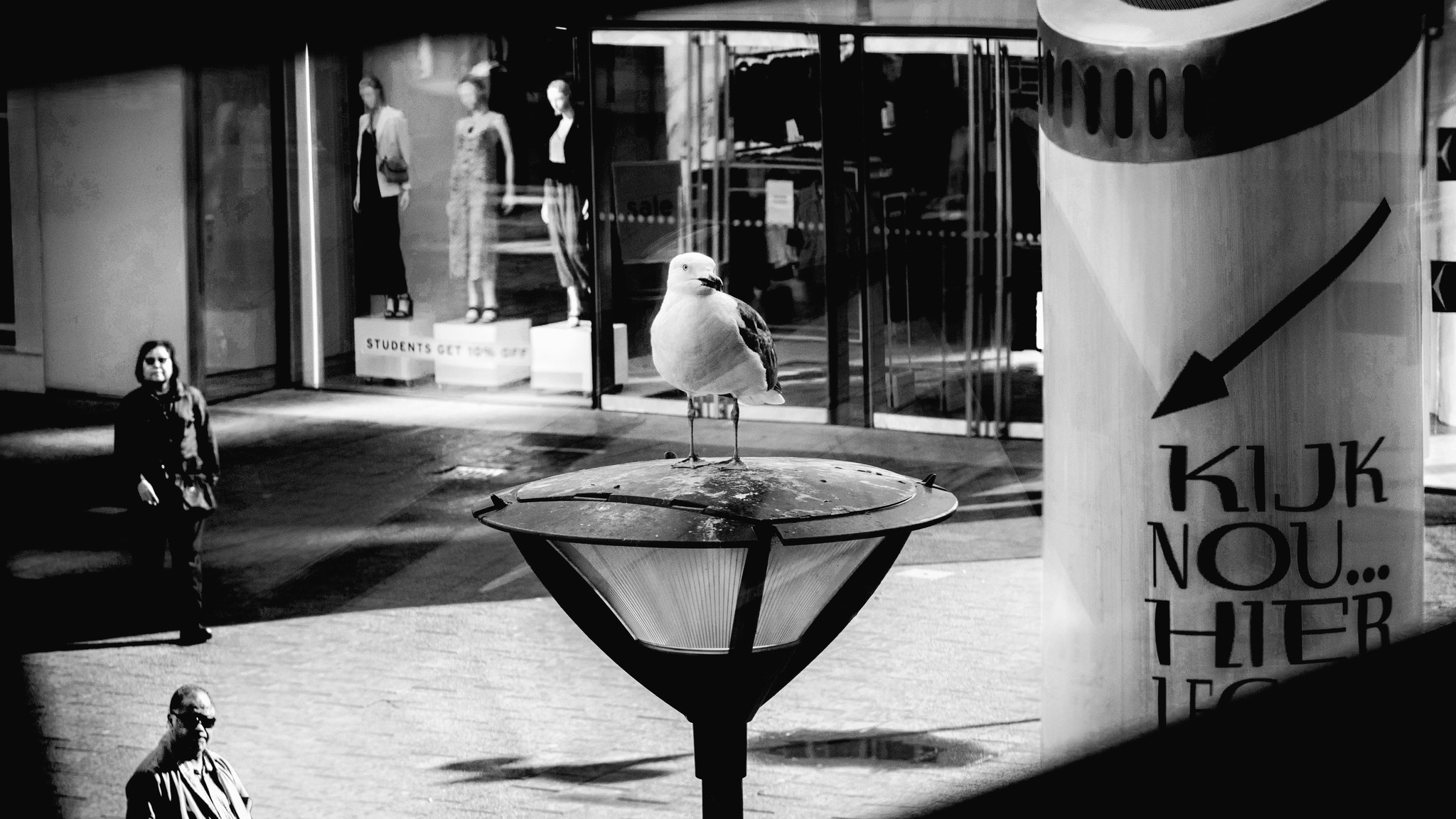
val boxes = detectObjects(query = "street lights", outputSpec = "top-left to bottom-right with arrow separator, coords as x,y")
473,392 -> 962,819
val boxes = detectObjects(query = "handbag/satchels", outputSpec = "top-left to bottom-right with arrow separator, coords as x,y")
171,473 -> 218,522
379,156 -> 410,183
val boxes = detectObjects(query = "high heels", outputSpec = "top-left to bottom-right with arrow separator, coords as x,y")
395,293 -> 413,318
384,296 -> 399,318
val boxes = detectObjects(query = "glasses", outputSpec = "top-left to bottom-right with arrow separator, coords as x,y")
172,710 -> 216,730
144,356 -> 168,365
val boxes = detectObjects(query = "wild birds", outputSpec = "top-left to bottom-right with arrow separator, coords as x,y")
647,250 -> 788,474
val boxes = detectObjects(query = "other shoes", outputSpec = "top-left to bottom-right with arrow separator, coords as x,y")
465,305 -> 482,323
482,302 -> 502,321
180,624 -> 212,644
566,306 -> 583,327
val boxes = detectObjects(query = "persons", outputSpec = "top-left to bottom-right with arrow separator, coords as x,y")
540,80 -> 593,327
353,75 -> 414,319
115,338 -> 221,646
123,685 -> 254,819
446,74 -> 516,323
